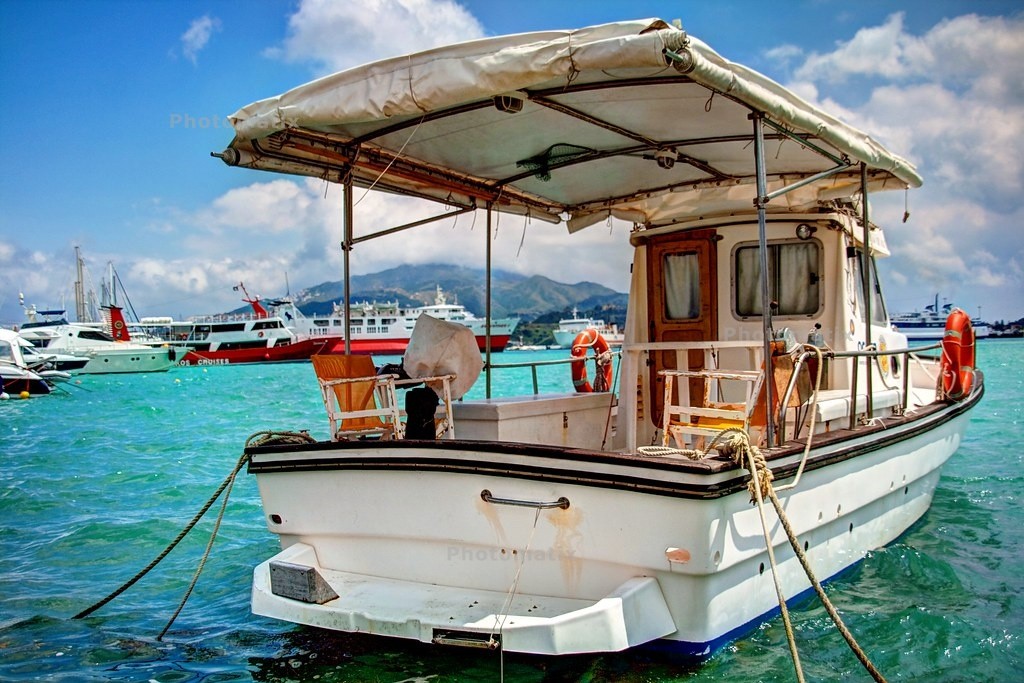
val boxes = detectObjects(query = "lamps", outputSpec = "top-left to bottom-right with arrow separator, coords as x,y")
491,90 -> 527,114
796,224 -> 817,240
654,147 -> 678,169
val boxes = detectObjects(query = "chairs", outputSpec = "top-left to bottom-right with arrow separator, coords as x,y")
310,354 -> 458,443
658,341 -> 784,450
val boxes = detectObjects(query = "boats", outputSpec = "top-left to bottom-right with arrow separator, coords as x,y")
889,294 -> 997,341
209,16 -> 985,663
551,305 -> 625,351
0,246 -> 523,399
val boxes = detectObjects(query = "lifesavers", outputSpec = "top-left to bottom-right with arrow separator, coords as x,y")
569,327 -> 614,393
940,306 -> 976,401
